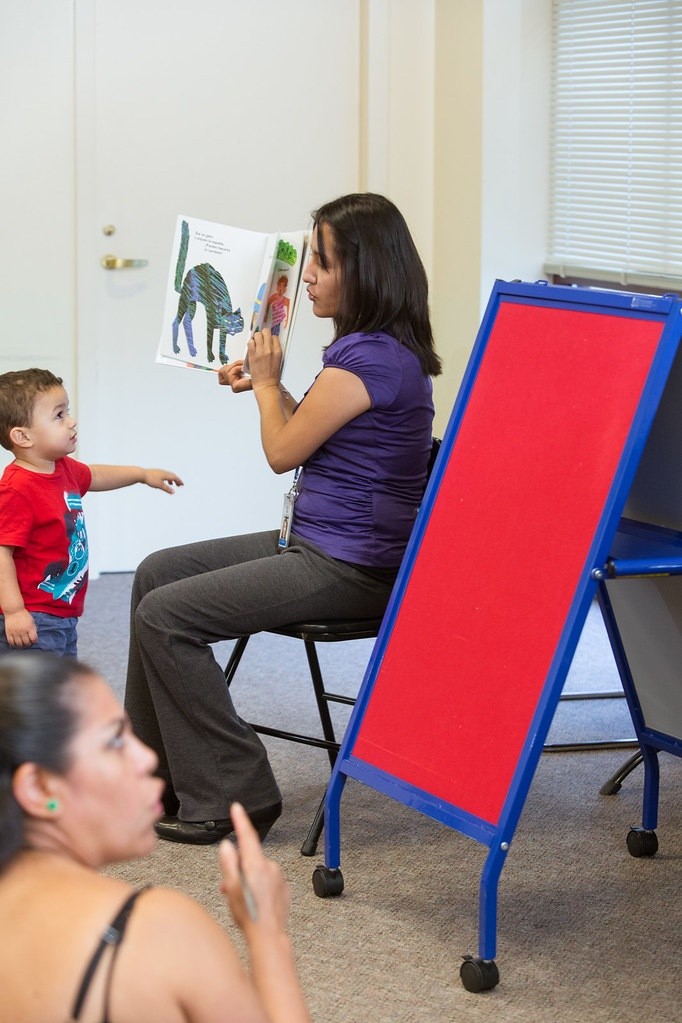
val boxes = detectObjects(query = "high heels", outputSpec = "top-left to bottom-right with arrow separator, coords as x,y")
153,800 -> 284,846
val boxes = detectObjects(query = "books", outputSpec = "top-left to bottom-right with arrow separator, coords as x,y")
158,213 -> 307,383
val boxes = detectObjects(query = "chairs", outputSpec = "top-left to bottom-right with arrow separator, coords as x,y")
217,432 -> 443,855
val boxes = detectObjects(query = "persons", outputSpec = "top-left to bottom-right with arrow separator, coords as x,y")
0,367 -> 185,664
0,647 -> 320,1023
123,193 -> 436,846
